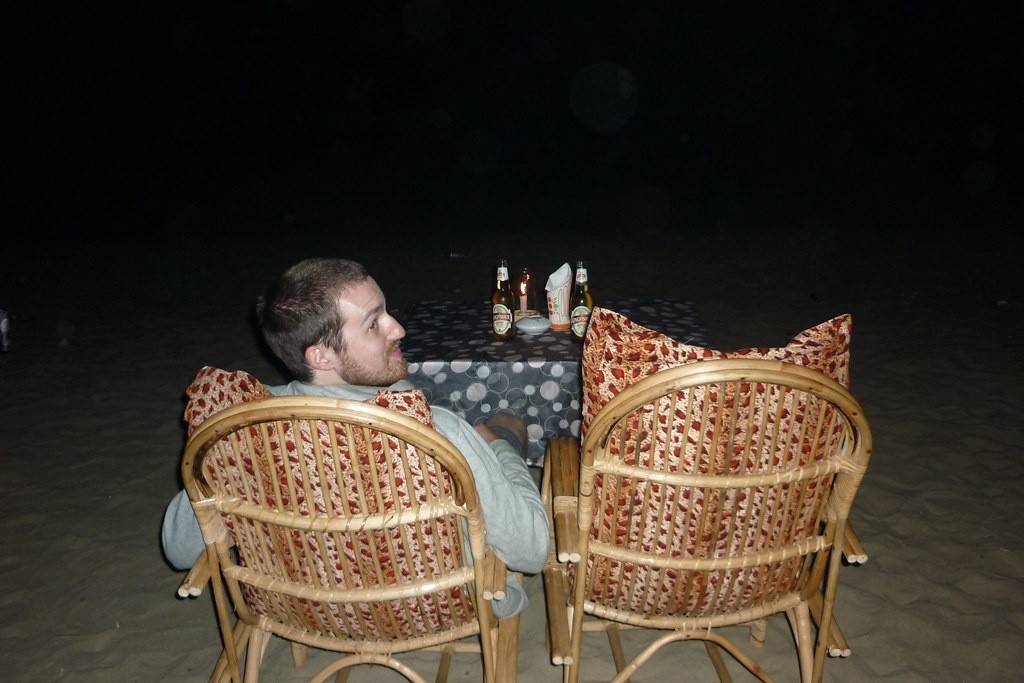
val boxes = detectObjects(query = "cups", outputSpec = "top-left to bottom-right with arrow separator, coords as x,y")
546,270 -> 572,330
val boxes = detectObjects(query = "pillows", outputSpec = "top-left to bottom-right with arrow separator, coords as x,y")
566,306 -> 852,618
184,366 -> 478,641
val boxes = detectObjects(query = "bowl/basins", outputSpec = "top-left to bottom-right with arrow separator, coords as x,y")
515,317 -> 552,336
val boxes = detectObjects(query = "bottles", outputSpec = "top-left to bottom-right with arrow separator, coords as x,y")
513,264 -> 536,309
493,257 -> 515,342
569,262 -> 592,342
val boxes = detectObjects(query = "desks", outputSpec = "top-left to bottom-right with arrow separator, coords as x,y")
398,295 -> 716,468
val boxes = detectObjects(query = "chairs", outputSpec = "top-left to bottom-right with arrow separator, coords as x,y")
178,396 -> 522,683
541,358 -> 873,683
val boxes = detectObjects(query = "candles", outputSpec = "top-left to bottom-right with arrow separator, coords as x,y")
519,282 -> 527,314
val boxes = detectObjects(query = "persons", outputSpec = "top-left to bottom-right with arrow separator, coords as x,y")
161,256 -> 550,620
